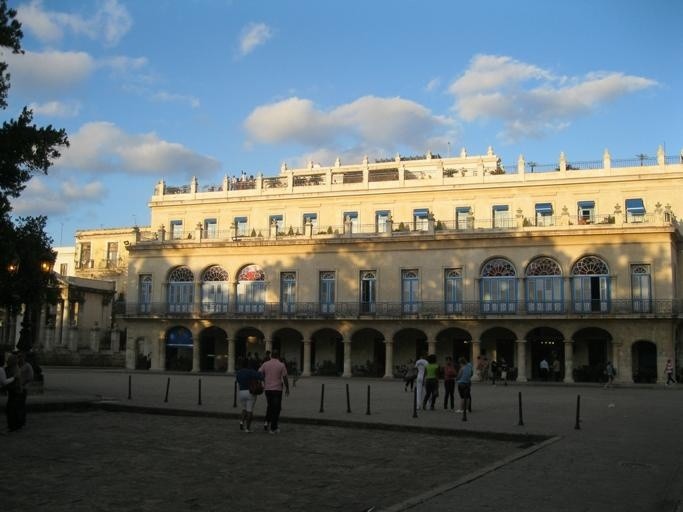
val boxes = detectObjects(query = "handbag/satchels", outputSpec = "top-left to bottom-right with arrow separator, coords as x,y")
248,380 -> 263,395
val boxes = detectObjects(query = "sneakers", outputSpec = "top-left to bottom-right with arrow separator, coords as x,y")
239,420 -> 243,430
263,421 -> 267,431
269,429 -> 280,434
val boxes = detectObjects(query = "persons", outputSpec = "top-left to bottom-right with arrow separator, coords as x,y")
231,172 -> 255,189
664,360 -> 677,387
1,352 -> 33,431
236,350 -> 290,434
404,354 -> 473,413
476,356 -> 508,386
550,357 -> 561,382
604,362 -> 613,389
540,358 -> 550,382
